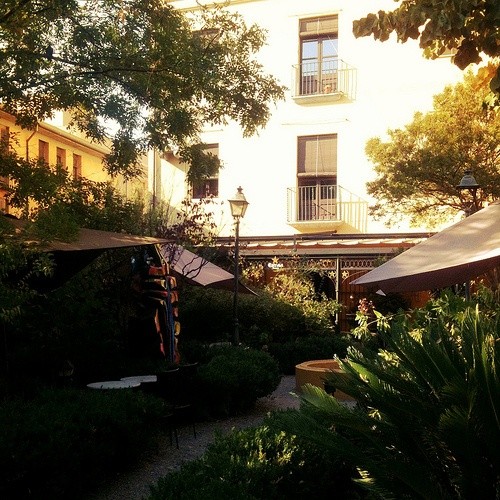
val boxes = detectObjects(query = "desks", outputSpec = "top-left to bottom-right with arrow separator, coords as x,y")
86,379 -> 142,393
120,373 -> 160,400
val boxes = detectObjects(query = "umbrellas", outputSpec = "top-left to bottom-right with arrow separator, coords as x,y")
154,237 -> 262,298
348,195 -> 500,292
0,213 -> 177,292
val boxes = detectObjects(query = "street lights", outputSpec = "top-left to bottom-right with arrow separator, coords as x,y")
226,184 -> 249,346
457,166 -> 482,301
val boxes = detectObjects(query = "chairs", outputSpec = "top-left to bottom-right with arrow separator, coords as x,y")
150,368 -> 179,456
170,361 -> 200,445
